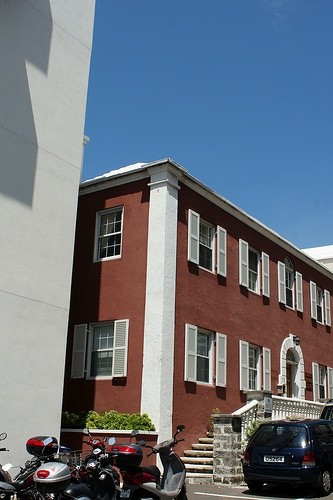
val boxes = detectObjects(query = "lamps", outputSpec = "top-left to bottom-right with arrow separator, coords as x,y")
293,336 -> 300,346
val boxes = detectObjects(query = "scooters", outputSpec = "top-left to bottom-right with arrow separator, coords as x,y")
105,424 -> 189,500
0,432 -> 58,500
31,427 -> 123,500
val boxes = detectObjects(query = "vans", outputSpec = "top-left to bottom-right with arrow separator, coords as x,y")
242,419 -> 333,498
